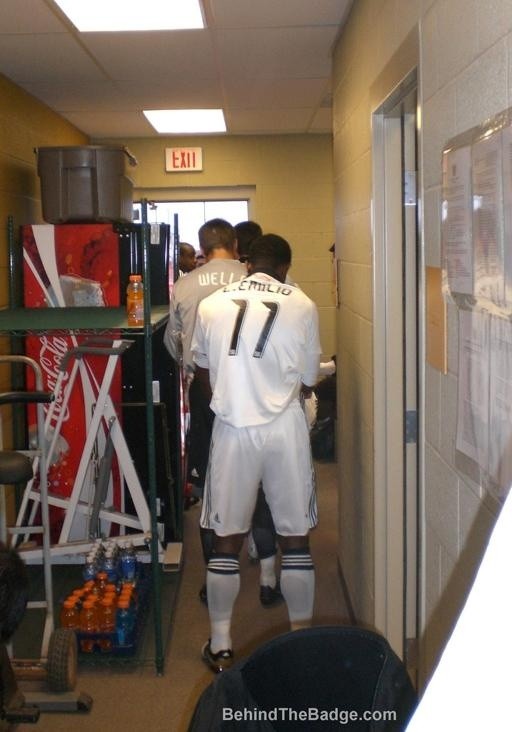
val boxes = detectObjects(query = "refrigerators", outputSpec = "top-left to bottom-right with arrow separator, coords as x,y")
20,221 -> 140,545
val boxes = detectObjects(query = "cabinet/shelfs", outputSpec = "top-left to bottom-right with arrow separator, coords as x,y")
1,204 -> 183,675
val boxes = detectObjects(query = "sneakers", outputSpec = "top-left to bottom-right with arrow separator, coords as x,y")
201,639 -> 233,672
259,582 -> 281,606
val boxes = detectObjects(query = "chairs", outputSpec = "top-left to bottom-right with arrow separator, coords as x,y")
188,625 -> 418,732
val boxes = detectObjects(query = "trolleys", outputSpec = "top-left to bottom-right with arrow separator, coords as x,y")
0,352 -> 82,694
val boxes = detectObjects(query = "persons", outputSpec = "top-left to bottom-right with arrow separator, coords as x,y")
164,216 -> 322,684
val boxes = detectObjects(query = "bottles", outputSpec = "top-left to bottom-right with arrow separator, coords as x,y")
126,274 -> 150,328
61,536 -> 138,656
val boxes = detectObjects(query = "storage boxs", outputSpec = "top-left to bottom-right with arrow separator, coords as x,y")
31,144 -> 142,226
